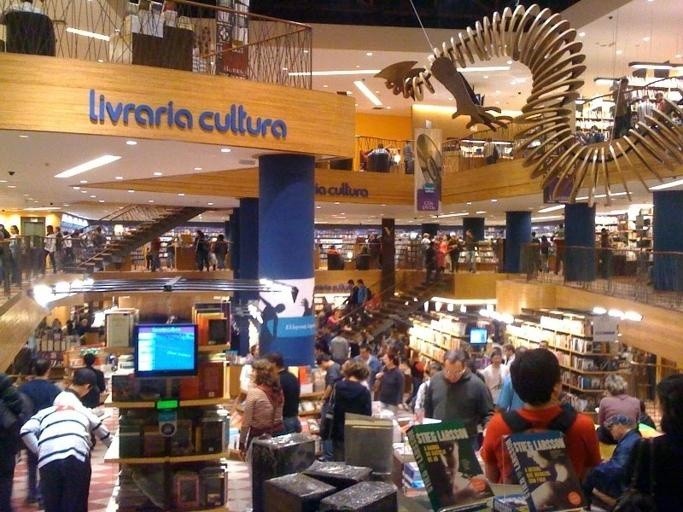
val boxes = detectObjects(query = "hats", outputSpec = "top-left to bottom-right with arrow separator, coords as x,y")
72,368 -> 100,395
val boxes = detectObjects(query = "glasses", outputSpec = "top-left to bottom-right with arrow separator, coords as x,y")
444,369 -> 466,376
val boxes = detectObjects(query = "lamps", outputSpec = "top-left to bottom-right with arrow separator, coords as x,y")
424,297 -> 515,324
557,0 -> 681,127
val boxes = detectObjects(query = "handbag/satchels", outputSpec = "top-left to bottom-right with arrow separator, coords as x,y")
318,382 -> 336,439
0,390 -> 40,452
607,437 -> 661,511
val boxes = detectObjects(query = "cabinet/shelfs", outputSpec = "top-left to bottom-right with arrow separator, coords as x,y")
594,216 -> 653,275
456,135 -> 512,177
72,223 -> 129,263
103,344 -> 234,511
533,222 -> 563,274
128,224 -> 228,270
297,389 -> 328,417
405,306 -> 653,425
64,343 -> 103,383
314,227 -> 507,272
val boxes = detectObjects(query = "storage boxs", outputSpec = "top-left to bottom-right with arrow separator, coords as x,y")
251,433 -> 400,512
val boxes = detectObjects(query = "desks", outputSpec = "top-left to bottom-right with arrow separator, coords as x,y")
108,23 -> 192,71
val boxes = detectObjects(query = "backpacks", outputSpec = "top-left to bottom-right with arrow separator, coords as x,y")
365,288 -> 372,301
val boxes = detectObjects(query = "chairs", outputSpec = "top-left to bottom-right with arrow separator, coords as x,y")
328,146 -> 393,172
4,12 -> 56,56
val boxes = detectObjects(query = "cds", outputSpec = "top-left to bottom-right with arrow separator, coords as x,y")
417,134 -> 442,201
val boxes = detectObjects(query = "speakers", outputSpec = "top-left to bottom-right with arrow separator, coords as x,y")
469,328 -> 488,344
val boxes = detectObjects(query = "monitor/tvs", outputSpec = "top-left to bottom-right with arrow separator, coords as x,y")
135,324 -> 198,377
